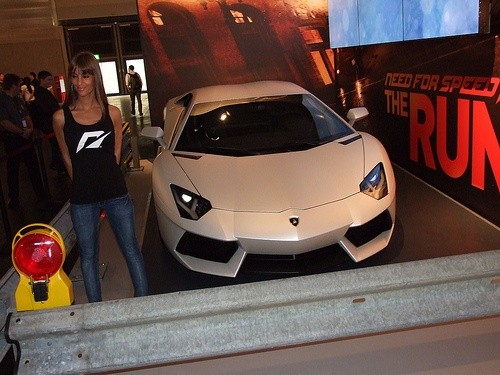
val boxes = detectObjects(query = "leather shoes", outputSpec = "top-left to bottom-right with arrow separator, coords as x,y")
36,193 -> 51,200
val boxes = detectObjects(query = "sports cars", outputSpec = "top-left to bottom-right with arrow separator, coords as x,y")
138,81 -> 397,278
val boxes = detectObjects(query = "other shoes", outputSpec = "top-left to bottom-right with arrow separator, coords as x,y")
9,197 -> 19,208
59,170 -> 66,175
49,164 -> 60,170
139,113 -> 143,115
130,111 -> 135,115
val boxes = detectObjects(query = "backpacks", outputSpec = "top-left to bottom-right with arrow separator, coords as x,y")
127,73 -> 141,92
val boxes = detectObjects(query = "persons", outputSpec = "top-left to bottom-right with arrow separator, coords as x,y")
29,72 -> 38,88
52,51 -> 149,303
21,77 -> 35,103
35,70 -> 67,170
125,66 -> 144,116
0,74 -> 63,210
0,73 -> 4,86
